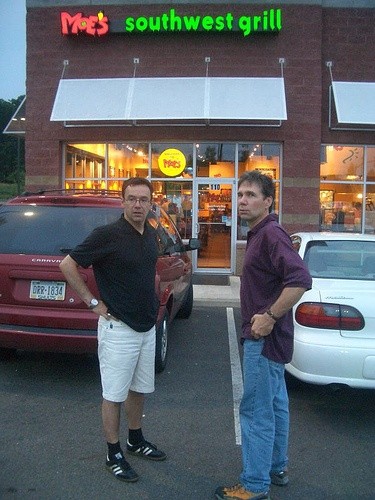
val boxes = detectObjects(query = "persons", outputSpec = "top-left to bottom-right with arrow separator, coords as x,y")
181,195 -> 193,217
59,178 -> 166,482
214,169 -> 312,499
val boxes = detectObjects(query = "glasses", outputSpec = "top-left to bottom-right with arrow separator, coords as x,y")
124,198 -> 151,206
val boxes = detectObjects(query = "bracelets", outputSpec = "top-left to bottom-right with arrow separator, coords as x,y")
266,309 -> 279,322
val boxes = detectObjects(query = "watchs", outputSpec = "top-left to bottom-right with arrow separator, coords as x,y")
88,298 -> 99,309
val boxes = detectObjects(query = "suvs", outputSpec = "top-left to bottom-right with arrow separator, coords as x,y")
0,189 -> 201,374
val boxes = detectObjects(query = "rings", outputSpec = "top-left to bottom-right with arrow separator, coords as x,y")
107,313 -> 112,316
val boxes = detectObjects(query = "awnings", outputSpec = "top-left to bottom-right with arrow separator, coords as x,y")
49,62 -> 288,127
2,97 -> 26,136
327,63 -> 375,132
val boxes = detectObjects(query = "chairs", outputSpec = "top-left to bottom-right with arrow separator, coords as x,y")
361,256 -> 375,277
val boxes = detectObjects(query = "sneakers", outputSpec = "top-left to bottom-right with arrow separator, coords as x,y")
106,454 -> 139,482
126,438 -> 166,460
271,471 -> 290,486
215,481 -> 270,500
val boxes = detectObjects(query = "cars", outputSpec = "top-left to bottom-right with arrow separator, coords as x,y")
285,233 -> 374,398
